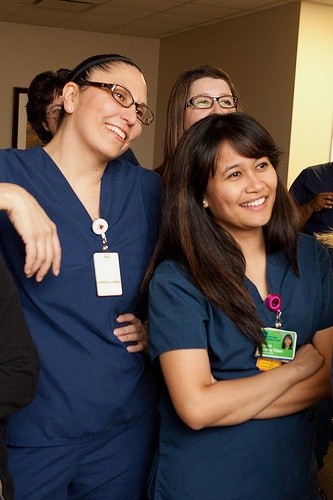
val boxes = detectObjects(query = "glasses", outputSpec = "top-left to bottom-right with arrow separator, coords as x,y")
44,105 -> 63,118
184,95 -> 238,108
77,80 -> 154,125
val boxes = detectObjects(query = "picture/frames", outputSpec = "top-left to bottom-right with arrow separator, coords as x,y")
12,87 -> 46,150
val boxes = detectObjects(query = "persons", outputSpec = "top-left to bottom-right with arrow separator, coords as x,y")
25,68 -> 141,167
287,161 -> 333,256
0,53 -> 164,500
136,113 -> 333,500
153,64 -> 245,178
